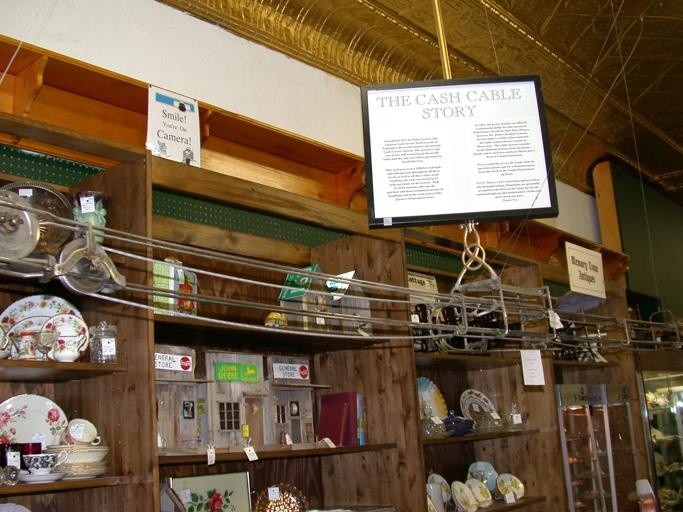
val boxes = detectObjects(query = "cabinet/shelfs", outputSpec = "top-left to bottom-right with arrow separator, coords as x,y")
0,36 -> 683,512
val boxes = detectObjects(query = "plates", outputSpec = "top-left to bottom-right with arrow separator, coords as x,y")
412,375 -> 448,423
0,294 -> 89,362
0,395 -> 109,483
459,388 -> 500,433
425,460 -> 525,512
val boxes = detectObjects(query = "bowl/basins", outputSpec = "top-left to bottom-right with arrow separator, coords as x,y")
2,182 -> 74,256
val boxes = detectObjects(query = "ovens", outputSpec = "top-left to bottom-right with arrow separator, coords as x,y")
554,382 -> 639,512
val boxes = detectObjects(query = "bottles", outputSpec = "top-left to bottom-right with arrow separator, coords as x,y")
89,320 -> 120,366
505,394 -> 528,430
419,405 -> 446,440
